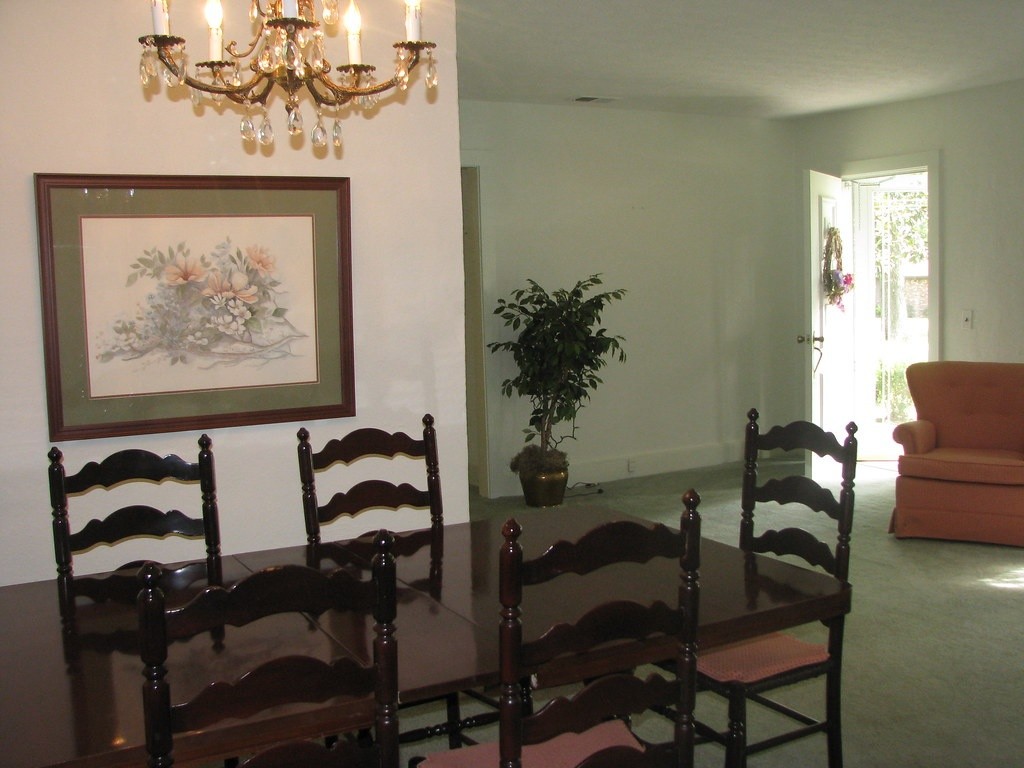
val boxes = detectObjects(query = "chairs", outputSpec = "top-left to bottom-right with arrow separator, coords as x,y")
298,413 -> 505,758
618,408 -> 858,768
408,488 -> 702,768
47,434 -> 239,768
136,529 -> 401,768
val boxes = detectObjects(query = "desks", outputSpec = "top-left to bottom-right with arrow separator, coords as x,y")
0,502 -> 852,768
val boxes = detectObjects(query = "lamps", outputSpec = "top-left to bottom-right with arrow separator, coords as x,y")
138,0 -> 439,147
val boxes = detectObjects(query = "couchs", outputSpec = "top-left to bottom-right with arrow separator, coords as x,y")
887,361 -> 1024,547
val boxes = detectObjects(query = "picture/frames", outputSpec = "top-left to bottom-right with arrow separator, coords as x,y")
35,172 -> 356,443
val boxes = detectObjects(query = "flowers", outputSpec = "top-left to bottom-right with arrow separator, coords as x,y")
821,226 -> 854,313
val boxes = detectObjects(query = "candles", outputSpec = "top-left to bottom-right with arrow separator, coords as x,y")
149,0 -> 421,65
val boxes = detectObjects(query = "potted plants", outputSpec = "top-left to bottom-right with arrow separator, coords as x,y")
485,273 -> 628,506
